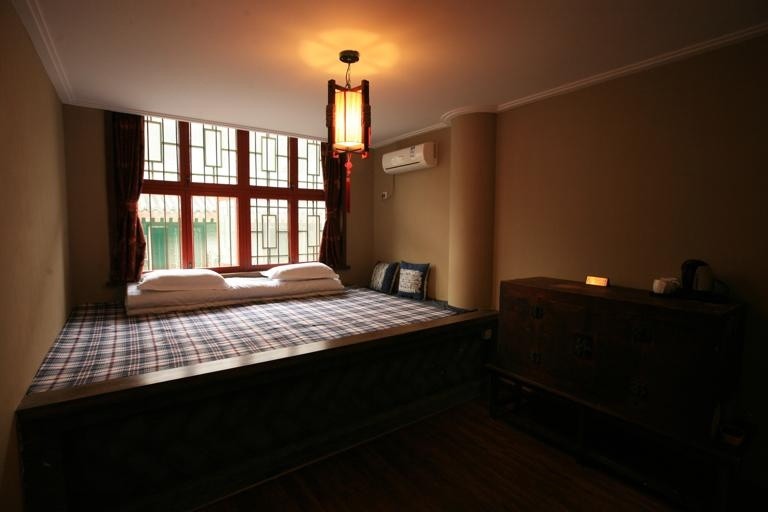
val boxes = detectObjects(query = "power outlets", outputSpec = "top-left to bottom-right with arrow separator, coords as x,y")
380,191 -> 387,200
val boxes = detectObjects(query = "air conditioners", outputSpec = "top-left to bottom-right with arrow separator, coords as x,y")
381,140 -> 439,174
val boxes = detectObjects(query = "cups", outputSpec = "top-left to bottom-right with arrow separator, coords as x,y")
653,276 -> 679,294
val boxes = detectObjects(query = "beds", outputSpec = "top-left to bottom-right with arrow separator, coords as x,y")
9,280 -> 496,511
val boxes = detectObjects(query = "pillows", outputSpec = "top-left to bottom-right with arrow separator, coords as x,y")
394,261 -> 432,301
369,261 -> 400,295
136,269 -> 231,292
259,262 -> 341,280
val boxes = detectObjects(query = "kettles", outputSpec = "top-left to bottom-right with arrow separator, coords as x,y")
679,259 -> 712,295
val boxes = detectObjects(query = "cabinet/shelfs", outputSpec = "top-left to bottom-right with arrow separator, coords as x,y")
488,276 -> 735,458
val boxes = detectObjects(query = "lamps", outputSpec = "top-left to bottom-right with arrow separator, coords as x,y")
324,49 -> 372,214
